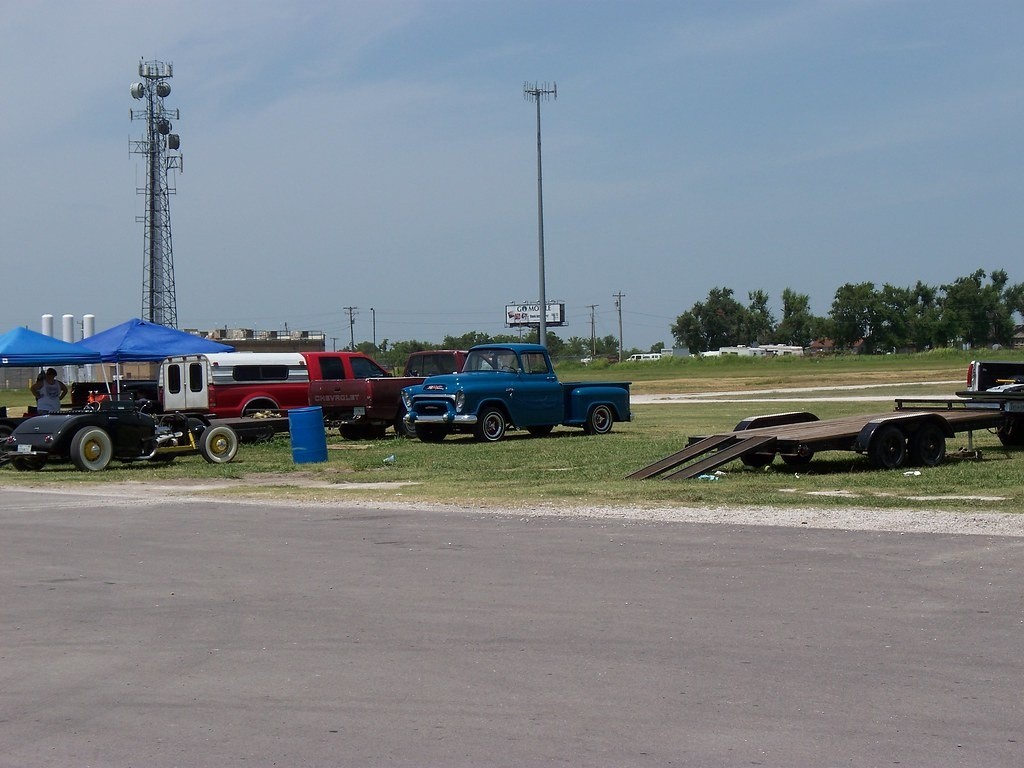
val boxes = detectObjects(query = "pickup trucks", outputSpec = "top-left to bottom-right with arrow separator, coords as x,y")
307,349 -> 504,439
627,354 -> 650,360
158,351 -> 394,442
401,344 -> 633,442
70,379 -> 158,414
640,354 -> 662,360
955,360 -> 1024,445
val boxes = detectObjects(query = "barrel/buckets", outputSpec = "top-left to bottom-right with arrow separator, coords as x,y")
288,406 -> 328,465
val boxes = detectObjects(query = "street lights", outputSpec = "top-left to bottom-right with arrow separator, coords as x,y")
371,307 -> 376,346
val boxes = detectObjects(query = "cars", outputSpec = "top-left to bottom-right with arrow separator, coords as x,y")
3,391 -> 239,472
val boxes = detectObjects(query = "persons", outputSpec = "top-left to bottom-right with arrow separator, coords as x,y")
30,368 -> 68,414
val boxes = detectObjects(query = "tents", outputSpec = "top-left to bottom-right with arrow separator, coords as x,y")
0,326 -> 115,412
71,317 -> 235,421
